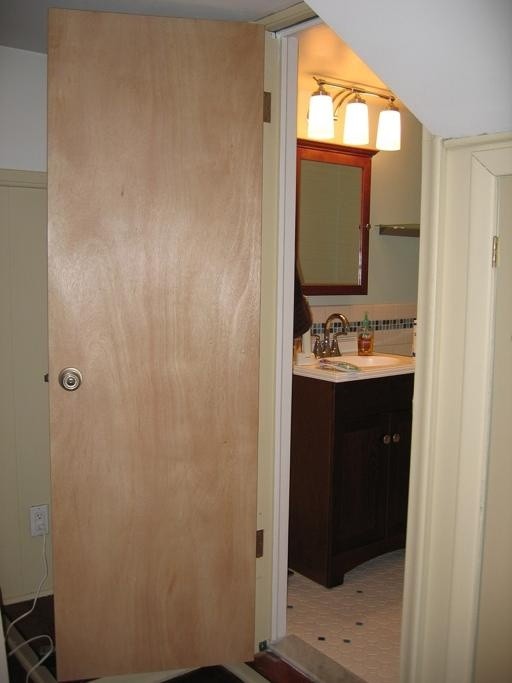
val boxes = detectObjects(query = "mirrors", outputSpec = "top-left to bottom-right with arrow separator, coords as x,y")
294,136 -> 380,296
290,375 -> 413,588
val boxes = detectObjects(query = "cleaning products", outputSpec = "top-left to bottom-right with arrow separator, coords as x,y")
357,312 -> 375,355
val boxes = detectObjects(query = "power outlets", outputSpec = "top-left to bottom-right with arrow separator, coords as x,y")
29,505 -> 49,537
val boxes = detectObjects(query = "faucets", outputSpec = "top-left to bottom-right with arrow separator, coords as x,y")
325,313 -> 351,350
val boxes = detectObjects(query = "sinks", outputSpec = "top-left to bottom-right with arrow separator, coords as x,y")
321,356 -> 399,368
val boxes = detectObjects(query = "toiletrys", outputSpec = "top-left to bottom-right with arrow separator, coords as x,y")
301,329 -> 312,354
293,336 -> 301,361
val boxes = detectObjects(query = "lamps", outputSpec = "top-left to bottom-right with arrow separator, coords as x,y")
306,77 -> 402,152
